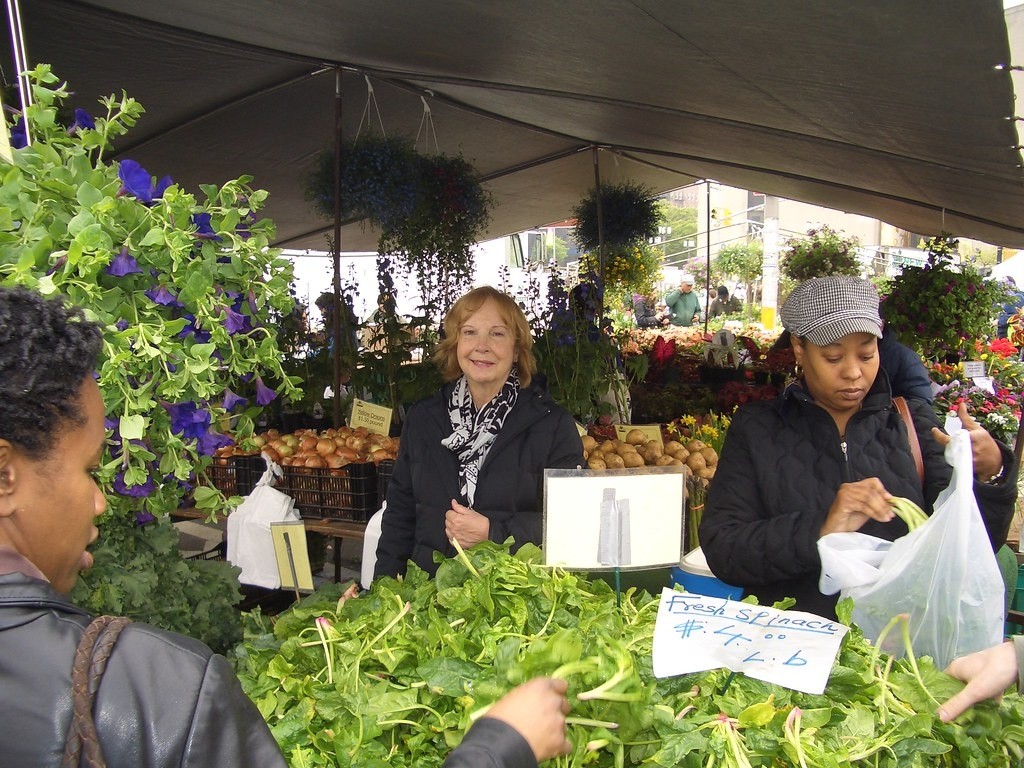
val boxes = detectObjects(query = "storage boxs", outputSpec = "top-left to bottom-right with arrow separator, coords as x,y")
278,462 -> 376,524
377,458 -> 395,510
200,454 -> 268,512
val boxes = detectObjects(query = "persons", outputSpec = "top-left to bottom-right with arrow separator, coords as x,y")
996,275 -> 1023,344
553,285 -> 632,427
368,286 -> 586,588
632,287 -> 665,329
710,285 -> 743,317
323,360 -> 353,400
937,634 -> 1024,725
666,277 -> 700,326
0,280 -> 571,768
315,293 -> 359,386
697,275 -> 1019,630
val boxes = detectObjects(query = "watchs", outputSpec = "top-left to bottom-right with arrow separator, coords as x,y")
981,464 -> 1006,487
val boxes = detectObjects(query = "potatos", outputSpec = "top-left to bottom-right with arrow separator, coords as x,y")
580,429 -> 718,486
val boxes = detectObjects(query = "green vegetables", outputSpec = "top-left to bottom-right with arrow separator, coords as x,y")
67,496 -> 1022,768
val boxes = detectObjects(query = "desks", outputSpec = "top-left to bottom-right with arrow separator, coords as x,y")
169,507 -> 368,584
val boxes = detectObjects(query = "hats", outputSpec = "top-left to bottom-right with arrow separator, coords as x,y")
718,286 -> 729,296
680,273 -> 695,286
779,275 -> 883,347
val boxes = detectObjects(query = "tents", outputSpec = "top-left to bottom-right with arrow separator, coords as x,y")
0,0 -> 1024,341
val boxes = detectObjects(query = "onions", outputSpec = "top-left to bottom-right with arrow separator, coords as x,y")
208,426 -> 400,517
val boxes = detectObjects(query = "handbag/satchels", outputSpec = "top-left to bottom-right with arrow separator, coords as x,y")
815,429 -> 1006,672
226,470 -> 302,589
